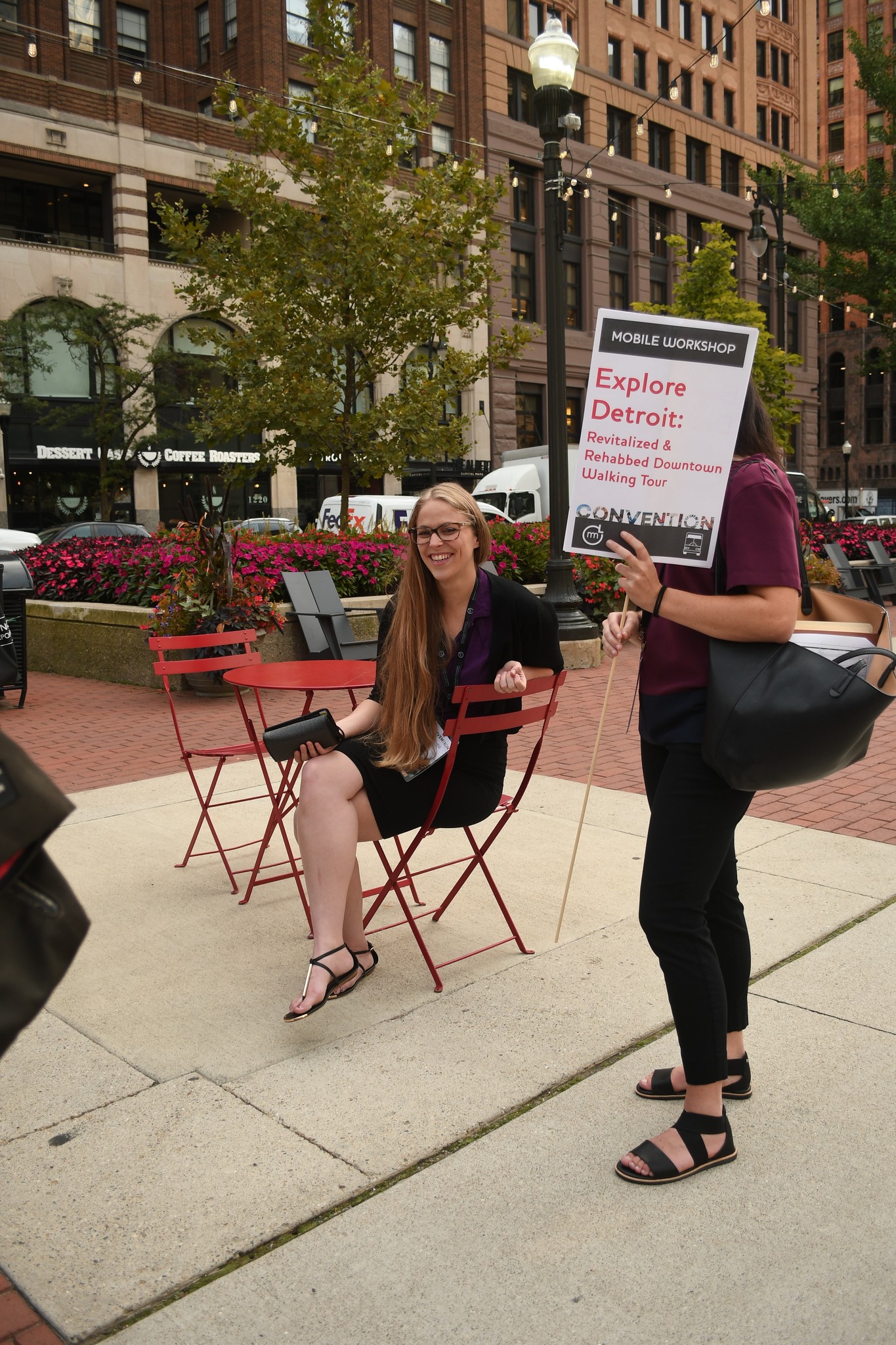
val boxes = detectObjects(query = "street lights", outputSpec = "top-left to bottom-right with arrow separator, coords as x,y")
526,8 -> 581,669
841,440 -> 852,519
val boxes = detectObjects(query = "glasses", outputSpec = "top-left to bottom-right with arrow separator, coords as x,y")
407,520 -> 471,544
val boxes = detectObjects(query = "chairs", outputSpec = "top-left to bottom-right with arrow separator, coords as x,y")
148,629 -> 298,895
866,541 -> 896,605
822,543 -> 886,610
363,670 -> 567,993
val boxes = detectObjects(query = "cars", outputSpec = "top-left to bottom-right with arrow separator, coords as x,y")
222,516 -> 303,537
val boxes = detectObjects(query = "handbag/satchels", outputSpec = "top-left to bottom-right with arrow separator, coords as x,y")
698,460 -> 895,791
0,736 -> 92,1056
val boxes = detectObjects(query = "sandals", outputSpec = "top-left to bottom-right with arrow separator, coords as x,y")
283,943 -> 357,1022
329,941 -> 379,1000
614,1108 -> 736,1182
635,1052 -> 751,1099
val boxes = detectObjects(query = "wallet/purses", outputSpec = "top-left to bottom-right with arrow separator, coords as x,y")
259,709 -> 348,761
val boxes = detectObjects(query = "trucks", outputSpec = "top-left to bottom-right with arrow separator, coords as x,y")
785,471 -> 836,524
469,443 -> 579,523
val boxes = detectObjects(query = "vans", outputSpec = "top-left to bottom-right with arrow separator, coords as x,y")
316,494 -> 515,537
836,515 -> 896,527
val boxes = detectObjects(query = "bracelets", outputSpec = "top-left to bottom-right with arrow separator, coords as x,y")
653,584 -> 669,618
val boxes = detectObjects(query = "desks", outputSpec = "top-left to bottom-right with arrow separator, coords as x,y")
222,661 -> 375,939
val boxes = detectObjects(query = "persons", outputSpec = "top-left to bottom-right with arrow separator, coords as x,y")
282,480 -> 563,1024
601,379 -> 800,1187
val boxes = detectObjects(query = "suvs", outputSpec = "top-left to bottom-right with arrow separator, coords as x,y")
36,521 -> 154,546
